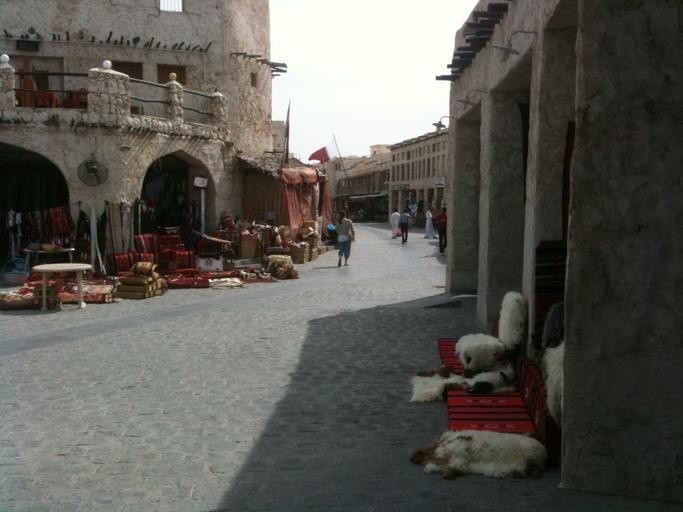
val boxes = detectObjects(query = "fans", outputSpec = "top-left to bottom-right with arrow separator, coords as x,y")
78,152 -> 109,186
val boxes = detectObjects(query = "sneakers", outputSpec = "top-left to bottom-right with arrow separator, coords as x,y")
424,236 -> 437,238
338,259 -> 348,267
393,236 -> 406,244
440,252 -> 444,255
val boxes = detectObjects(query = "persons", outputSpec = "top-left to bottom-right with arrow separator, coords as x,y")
389,208 -> 400,238
435,207 -> 447,256
335,210 -> 354,267
398,208 -> 410,244
423,208 -> 437,239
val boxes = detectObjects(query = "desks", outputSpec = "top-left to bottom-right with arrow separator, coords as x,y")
23,246 -> 92,310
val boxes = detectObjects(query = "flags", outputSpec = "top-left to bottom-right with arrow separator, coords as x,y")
308,146 -> 330,164
